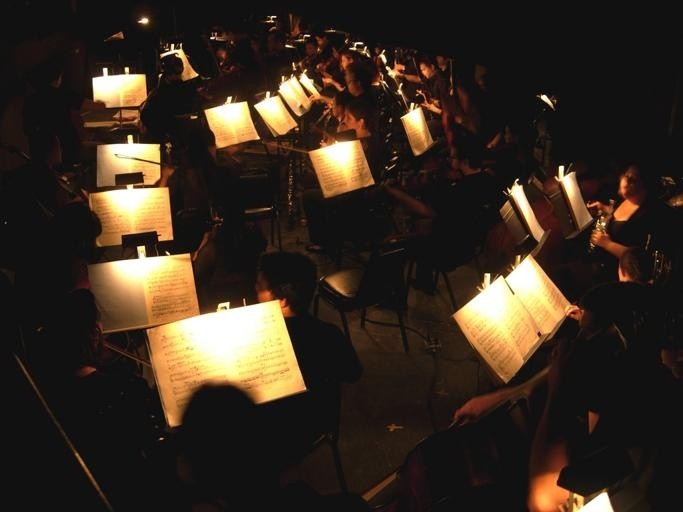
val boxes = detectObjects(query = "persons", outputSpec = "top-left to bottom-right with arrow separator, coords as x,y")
1,2 -> 683,511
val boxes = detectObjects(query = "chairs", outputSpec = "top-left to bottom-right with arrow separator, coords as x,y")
237,172 -> 464,365
